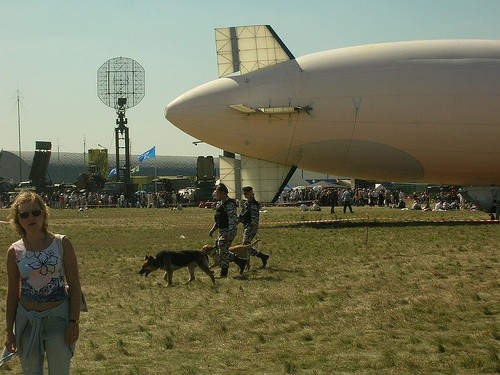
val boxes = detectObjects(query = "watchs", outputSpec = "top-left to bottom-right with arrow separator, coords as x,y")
68,320 -> 80,324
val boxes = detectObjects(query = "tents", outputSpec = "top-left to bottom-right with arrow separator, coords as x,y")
285,181 -> 348,190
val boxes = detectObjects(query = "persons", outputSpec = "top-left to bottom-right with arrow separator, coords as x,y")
209,184 -> 248,278
4,192 -> 82,375
0,190 -> 194,209
282,186 -> 466,213
235,186 -> 269,267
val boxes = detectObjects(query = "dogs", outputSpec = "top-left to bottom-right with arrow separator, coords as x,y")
201,239 -> 262,272
138,249 -> 215,287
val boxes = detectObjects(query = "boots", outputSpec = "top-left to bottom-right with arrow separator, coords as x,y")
215,269 -> 228,278
258,252 -> 268,266
236,257 -> 247,275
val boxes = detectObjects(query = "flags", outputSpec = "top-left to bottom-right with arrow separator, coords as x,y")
139,148 -> 155,162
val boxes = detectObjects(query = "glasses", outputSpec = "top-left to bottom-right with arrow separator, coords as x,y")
17,209 -> 43,218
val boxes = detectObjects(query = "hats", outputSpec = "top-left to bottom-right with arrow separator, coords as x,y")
242,187 -> 254,191
219,182 -> 228,194
